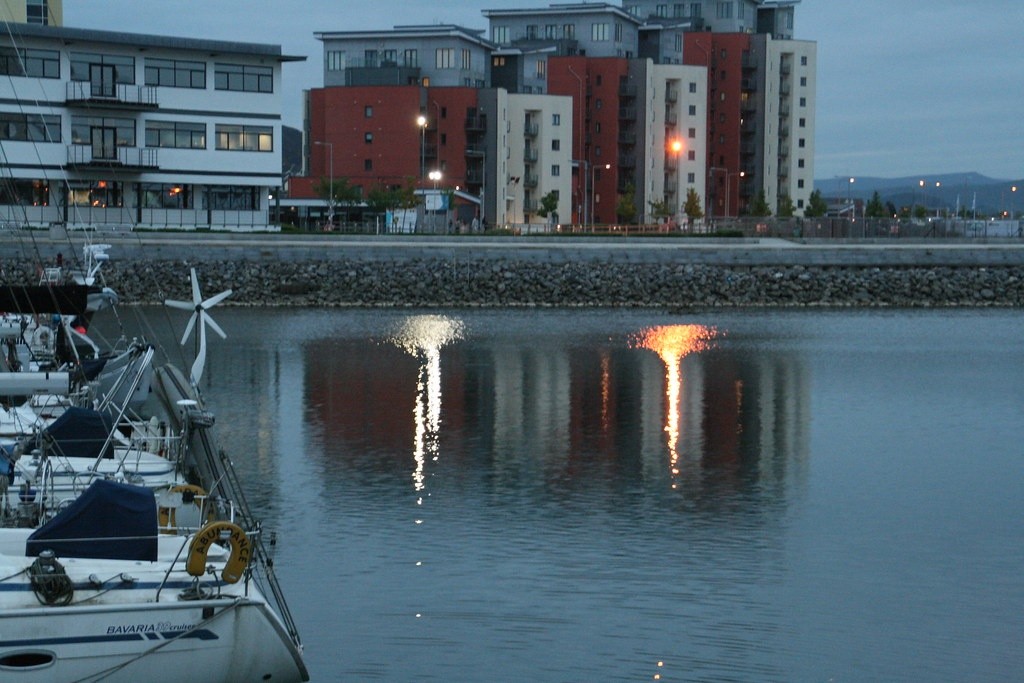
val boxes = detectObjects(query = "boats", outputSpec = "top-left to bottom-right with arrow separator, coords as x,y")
1,0 -> 311,683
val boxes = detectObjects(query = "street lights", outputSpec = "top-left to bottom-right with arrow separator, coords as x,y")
417,116 -> 426,197
428,171 -> 441,234
710,167 -> 745,218
671,140 -> 684,221
848,177 -> 855,238
592,164 -> 610,232
468,150 -> 484,218
315,142 -> 333,234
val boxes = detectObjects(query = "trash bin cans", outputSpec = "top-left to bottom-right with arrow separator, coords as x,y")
48,221 -> 67,239
